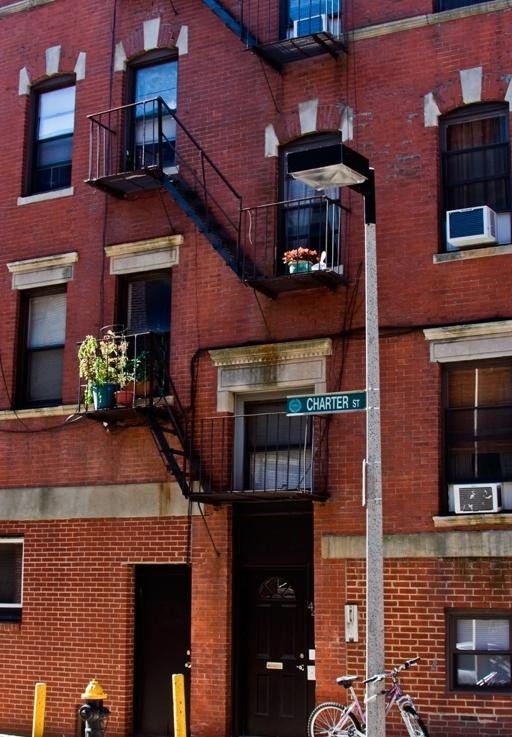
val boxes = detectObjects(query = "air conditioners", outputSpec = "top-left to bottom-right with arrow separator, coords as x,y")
294,14 -> 331,38
446,205 -> 499,248
453,483 -> 501,514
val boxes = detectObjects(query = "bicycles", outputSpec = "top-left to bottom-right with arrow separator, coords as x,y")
307,656 -> 430,737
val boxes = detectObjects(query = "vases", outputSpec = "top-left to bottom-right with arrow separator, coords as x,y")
288,259 -> 312,274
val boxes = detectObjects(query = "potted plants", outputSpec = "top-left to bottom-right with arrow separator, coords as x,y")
125,351 -> 151,397
78,330 -> 117,409
97,330 -> 129,391
114,372 -> 138,406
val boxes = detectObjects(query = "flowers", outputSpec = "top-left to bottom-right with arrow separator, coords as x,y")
282,247 -> 319,265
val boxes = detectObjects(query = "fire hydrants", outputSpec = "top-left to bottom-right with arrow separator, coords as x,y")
78,678 -> 111,737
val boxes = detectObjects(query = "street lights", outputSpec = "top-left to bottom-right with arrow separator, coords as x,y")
286,143 -> 385,737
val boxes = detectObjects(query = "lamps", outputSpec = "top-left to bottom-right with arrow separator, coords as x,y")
344,600 -> 358,644
